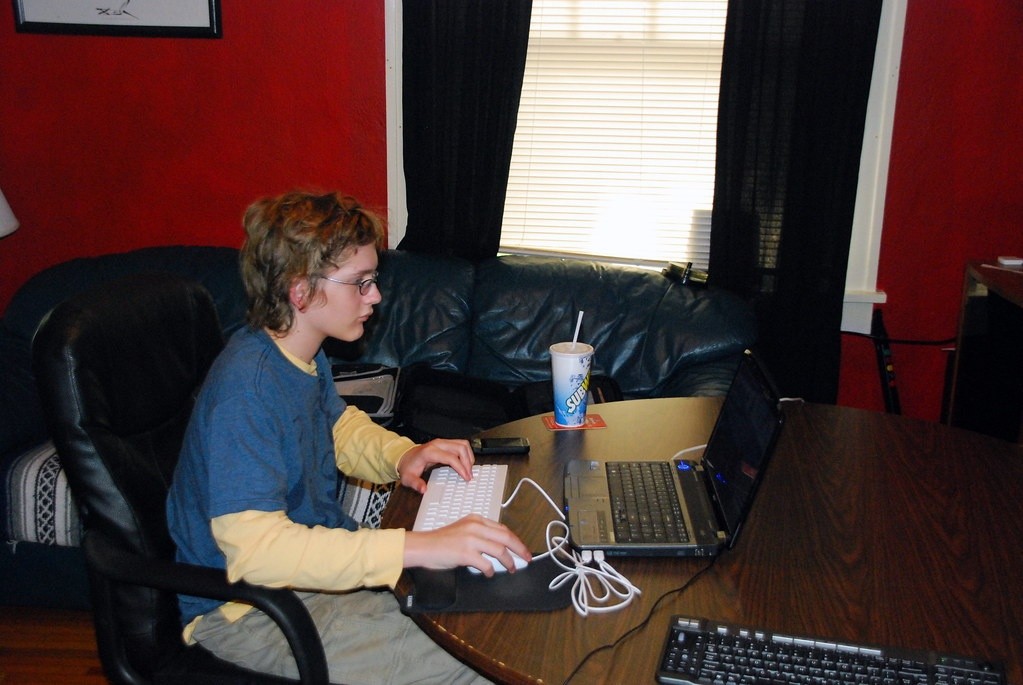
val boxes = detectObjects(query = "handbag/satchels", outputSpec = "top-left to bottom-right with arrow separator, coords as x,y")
392,361 -> 510,444
511,375 -> 623,421
330,363 -> 401,430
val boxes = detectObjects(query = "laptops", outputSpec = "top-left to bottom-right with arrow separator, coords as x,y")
562,349 -> 784,559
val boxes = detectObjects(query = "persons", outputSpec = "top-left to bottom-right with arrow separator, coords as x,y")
166,194 -> 531,685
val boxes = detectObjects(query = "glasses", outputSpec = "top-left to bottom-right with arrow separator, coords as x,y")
297,272 -> 379,296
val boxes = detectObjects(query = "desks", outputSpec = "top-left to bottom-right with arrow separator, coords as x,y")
947,261 -> 1023,425
386,395 -> 1023,685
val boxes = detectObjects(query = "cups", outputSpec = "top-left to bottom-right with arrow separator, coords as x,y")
550,342 -> 594,428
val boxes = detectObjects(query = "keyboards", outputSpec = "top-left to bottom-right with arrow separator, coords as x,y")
411,463 -> 509,532
654,614 -> 1008,685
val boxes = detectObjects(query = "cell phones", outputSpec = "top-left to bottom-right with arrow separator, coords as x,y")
473,438 -> 531,454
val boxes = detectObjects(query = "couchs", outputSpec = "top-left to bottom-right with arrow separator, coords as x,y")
28,278 -> 329,684
0,244 -> 761,616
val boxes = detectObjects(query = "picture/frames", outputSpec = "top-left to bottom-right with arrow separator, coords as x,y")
12,0 -> 223,40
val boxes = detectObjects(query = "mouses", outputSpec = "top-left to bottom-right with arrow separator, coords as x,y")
466,546 -> 528,574
